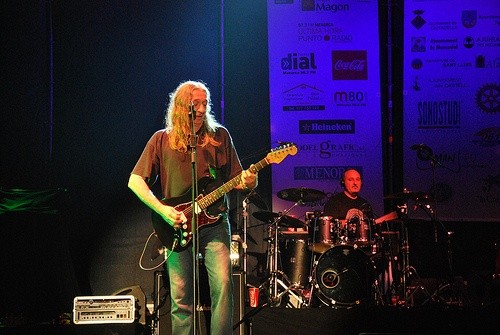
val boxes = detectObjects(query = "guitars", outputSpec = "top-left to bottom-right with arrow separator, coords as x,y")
152,143 -> 298,253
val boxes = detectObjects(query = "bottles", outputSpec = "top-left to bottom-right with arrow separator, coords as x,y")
230,235 -> 240,265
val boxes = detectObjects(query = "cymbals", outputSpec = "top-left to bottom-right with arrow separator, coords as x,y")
282,229 -> 309,237
277,187 -> 329,203
377,229 -> 402,237
385,189 -> 424,204
252,211 -> 304,228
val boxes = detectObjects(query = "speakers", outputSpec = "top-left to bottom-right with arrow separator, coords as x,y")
153,273 -> 247,335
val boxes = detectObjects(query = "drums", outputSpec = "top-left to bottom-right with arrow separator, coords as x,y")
313,246 -> 379,309
279,241 -> 310,289
347,219 -> 373,249
309,215 -> 339,252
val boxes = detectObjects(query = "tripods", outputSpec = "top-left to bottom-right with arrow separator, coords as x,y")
255,198 -> 469,310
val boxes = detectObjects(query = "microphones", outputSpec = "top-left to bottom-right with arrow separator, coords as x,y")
403,188 -> 419,194
408,144 -> 423,152
189,102 -> 196,118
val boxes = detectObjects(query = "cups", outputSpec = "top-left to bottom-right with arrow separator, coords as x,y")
249,288 -> 259,307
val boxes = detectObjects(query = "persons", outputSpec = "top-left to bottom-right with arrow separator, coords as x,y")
128,79 -> 258,335
324,168 -> 374,221
